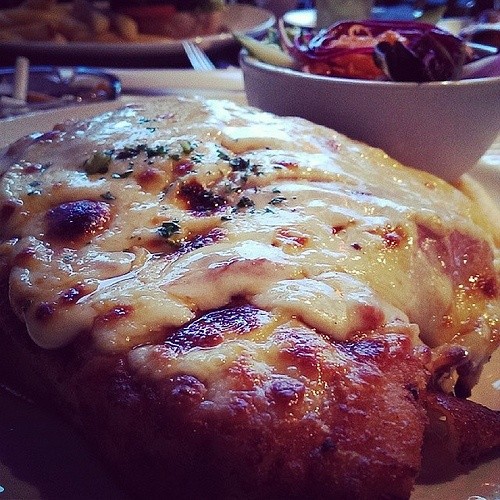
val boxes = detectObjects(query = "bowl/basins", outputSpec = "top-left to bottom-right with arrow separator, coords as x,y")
240,44 -> 500,181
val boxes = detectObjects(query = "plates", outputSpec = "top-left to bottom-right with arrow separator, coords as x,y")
1,100 -> 500,500
283,9 -> 316,27
0,2 -> 275,56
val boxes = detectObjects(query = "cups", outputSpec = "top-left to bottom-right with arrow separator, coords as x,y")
316,0 -> 373,31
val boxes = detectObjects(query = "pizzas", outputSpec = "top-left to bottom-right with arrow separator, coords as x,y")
1,98 -> 491,500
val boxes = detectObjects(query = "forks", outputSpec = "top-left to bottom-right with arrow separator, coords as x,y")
181,40 -> 216,71
